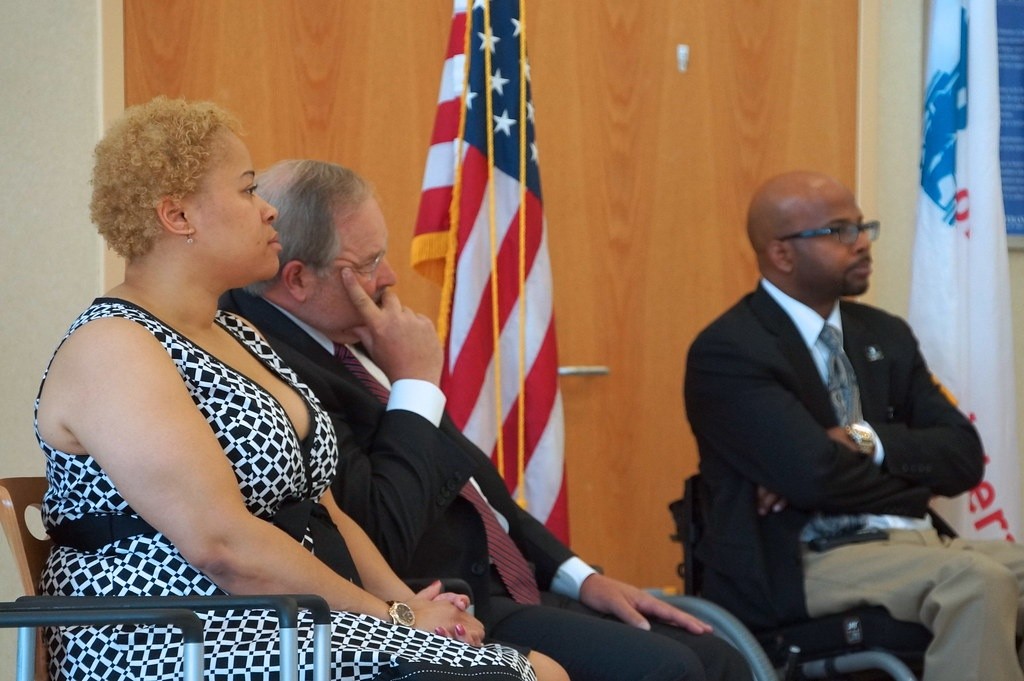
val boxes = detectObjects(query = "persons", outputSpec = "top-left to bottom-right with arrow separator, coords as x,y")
683,167 -> 1024,681
33,99 -> 577,681
217,159 -> 756,681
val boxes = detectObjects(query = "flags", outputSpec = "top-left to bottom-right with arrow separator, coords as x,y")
411,0 -> 573,552
909,1 -> 1024,547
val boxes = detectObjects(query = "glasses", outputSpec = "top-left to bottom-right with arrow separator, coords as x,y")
777,217 -> 880,245
314,248 -> 390,278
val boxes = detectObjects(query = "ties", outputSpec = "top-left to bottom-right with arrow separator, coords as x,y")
820,325 -> 862,427
332,341 -> 540,605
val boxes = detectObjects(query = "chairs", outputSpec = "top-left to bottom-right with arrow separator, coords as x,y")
0,476 -> 778,681
666,472 -> 1024,681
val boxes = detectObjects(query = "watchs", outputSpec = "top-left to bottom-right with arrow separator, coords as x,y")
385,600 -> 415,628
845,424 -> 876,454
